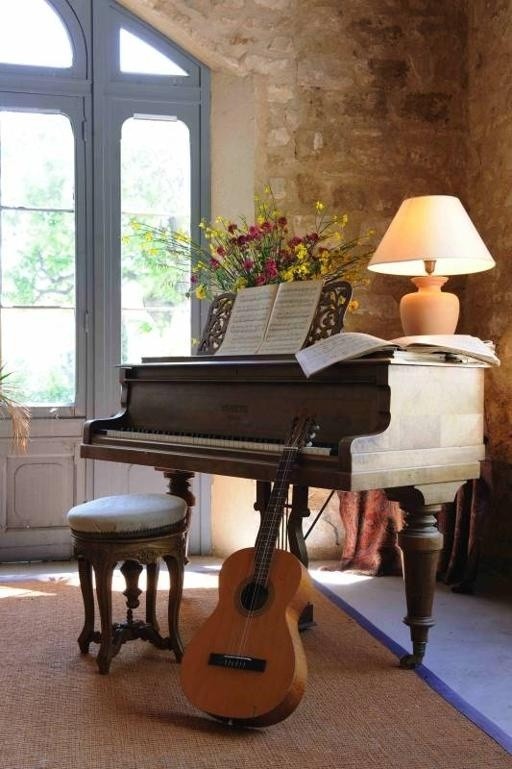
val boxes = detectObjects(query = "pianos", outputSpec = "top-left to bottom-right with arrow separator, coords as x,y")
79,282 -> 492,668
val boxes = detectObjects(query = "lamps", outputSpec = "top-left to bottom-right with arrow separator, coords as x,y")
368,196 -> 496,336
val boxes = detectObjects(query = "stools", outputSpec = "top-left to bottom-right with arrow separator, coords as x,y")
67,492 -> 191,675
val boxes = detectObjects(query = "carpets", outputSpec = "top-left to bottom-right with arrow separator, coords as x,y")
0,568 -> 512,767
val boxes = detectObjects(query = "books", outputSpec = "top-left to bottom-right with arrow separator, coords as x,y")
211,278 -> 502,379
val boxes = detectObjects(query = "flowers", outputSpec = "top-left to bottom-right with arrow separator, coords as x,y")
118,184 -> 376,314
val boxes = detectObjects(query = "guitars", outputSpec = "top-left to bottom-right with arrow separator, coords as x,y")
181,412 -> 321,729
